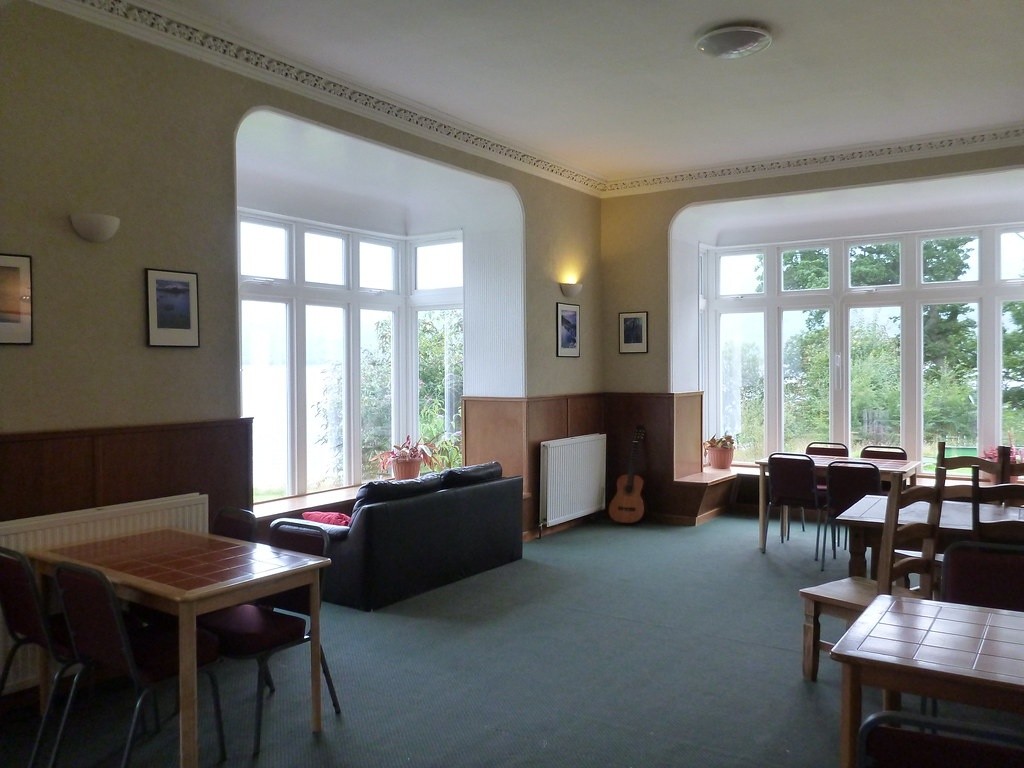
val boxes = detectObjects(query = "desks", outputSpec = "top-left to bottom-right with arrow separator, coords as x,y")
828,594 -> 1024,768
834,494 -> 1024,578
756,454 -> 922,553
24,526 -> 331,768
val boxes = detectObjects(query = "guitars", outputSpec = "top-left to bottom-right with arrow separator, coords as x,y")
608,425 -> 646,524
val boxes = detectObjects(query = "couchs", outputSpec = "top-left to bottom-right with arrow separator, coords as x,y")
269,461 -> 523,613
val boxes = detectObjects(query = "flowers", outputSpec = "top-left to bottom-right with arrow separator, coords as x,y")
370,430 -> 445,471
703,431 -> 736,457
980,430 -> 1024,464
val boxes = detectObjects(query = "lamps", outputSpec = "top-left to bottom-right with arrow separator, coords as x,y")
70,214 -> 120,244
696,25 -> 773,60
559,283 -> 582,298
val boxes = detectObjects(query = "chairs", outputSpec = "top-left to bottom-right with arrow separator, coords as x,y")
761,441 -> 1024,768
0,505 -> 342,768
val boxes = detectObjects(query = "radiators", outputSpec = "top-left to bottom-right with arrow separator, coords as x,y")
540,433 -> 606,538
0,494 -> 211,695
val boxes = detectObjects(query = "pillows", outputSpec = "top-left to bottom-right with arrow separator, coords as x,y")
302,511 -> 350,526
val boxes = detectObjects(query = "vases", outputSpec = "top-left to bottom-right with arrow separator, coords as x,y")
707,448 -> 734,469
393,457 -> 421,481
989,473 -> 1018,485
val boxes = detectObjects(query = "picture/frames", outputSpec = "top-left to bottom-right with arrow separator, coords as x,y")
619,310 -> 648,354
556,302 -> 580,358
1,254 -> 33,346
144,269 -> 199,348
